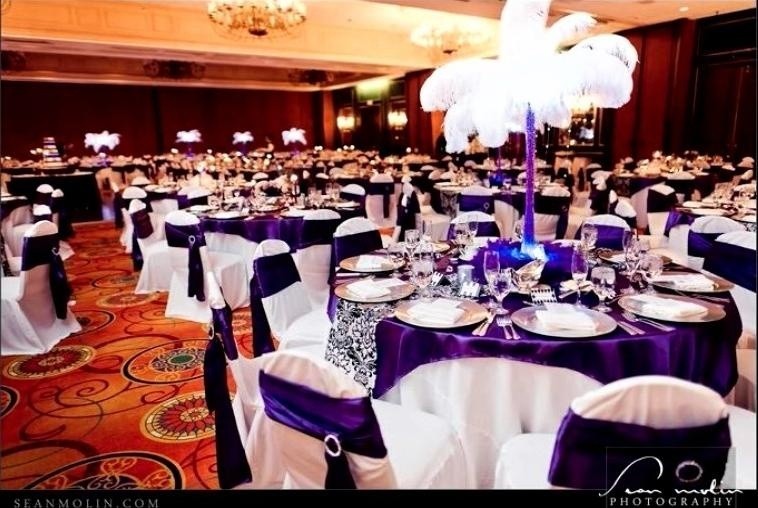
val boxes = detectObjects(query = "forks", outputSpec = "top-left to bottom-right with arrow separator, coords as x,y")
620,310 -> 676,334
496,314 -> 521,340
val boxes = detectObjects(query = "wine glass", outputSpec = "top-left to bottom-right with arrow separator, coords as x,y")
374,214 -> 512,313
194,174 -> 349,209
711,175 -> 757,223
571,220 -> 663,311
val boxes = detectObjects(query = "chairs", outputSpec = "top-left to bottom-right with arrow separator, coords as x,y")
1,155 -> 756,490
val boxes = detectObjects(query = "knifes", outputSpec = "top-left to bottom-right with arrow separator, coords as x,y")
471,312 -> 498,337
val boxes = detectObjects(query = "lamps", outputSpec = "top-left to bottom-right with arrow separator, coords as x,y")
207,1 -> 307,40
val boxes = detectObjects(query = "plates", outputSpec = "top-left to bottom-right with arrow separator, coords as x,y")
515,176 -> 566,193
277,213 -> 302,218
618,293 -> 728,323
597,250 -> 673,262
339,254 -> 407,273
203,214 -> 248,219
680,199 -> 723,216
509,304 -> 619,338
333,277 -> 414,304
396,296 -> 491,332
646,272 -> 735,292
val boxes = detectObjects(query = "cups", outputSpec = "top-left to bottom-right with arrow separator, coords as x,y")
448,167 -> 512,195
